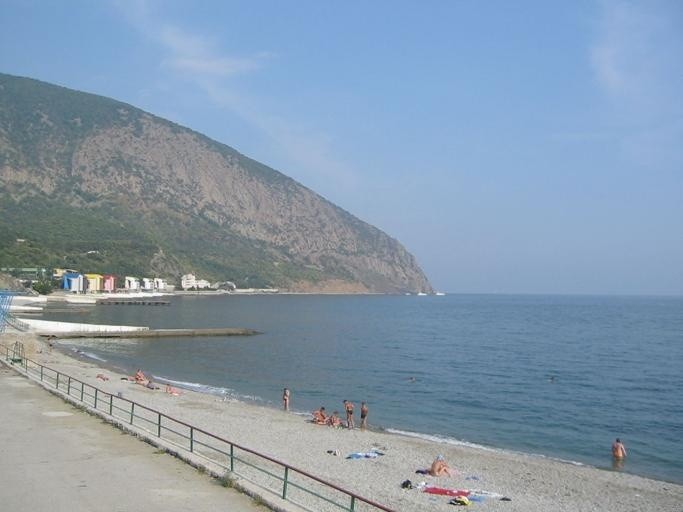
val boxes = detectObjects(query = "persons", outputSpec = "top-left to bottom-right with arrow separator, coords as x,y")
612,437 -> 627,460
409,377 -> 416,382
282,388 -> 290,412
430,454 -> 453,478
36,337 -> 179,396
311,399 -> 369,432
